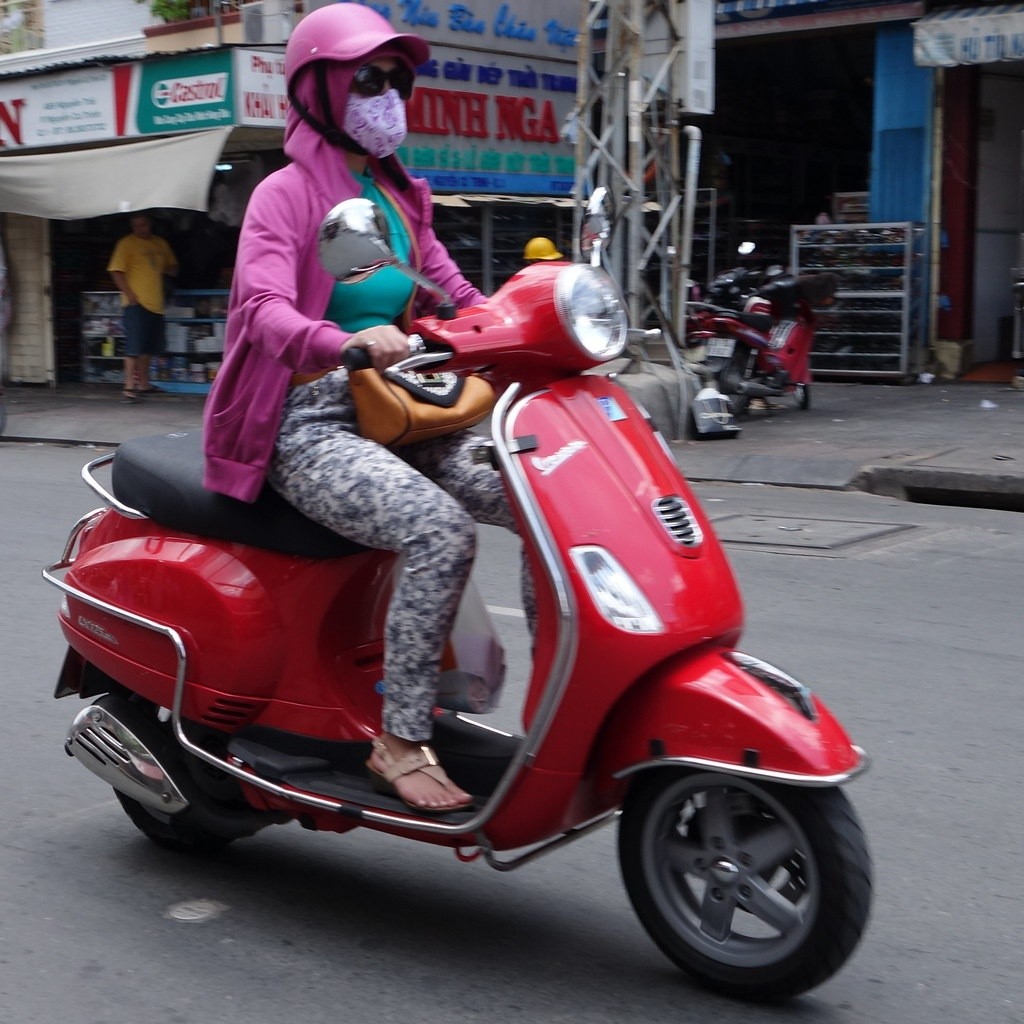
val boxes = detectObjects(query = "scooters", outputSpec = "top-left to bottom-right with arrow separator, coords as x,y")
36,186 -> 877,999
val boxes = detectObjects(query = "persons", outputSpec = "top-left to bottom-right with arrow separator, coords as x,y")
201,5 -> 537,817
109,213 -> 178,401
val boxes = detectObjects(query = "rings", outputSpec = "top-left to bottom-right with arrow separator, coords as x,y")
366,339 -> 379,348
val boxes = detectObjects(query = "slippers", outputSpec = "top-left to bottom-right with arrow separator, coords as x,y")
121,384 -> 165,399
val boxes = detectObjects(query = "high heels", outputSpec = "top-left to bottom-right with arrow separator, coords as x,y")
365,735 -> 476,813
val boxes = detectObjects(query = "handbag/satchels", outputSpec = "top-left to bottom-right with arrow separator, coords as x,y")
690,398 -> 743,441
344,346 -> 499,446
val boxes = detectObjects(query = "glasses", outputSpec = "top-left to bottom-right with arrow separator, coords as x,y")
352,63 -> 414,99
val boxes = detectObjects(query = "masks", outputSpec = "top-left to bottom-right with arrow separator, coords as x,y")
343,88 -> 408,160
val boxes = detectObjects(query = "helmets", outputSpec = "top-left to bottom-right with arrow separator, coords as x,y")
285,2 -> 430,92
524,236 -> 563,260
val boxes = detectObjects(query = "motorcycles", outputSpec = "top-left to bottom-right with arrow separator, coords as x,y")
680,240 -> 836,421
